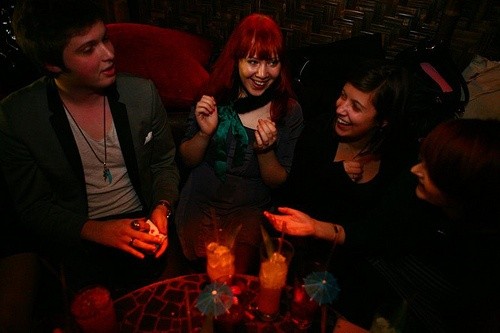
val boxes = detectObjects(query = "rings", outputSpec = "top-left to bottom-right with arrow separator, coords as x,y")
129,237 -> 134,246
131,219 -> 140,230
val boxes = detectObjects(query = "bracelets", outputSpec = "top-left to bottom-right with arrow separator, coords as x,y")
332,223 -> 339,249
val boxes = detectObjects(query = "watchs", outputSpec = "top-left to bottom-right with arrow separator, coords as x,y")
159,201 -> 174,219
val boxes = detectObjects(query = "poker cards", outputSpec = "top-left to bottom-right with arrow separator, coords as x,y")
145,220 -> 166,251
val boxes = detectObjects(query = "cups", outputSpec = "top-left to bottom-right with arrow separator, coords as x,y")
290,261 -> 329,330
204,236 -> 236,285
70,287 -> 115,332
254,237 -> 295,321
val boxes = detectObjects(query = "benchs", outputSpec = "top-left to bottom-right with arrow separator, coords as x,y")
106,23 -> 212,129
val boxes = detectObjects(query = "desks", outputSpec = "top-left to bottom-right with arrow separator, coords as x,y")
54,273 -> 368,333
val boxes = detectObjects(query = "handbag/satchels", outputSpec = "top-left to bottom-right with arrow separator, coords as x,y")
280,47 -> 322,121
406,38 -> 470,121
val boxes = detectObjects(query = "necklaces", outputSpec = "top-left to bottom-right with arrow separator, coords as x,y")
61,93 -> 112,184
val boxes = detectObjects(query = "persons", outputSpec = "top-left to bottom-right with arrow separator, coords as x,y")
174,12 -> 303,274
0,0 -> 182,302
256,63 -> 500,333
